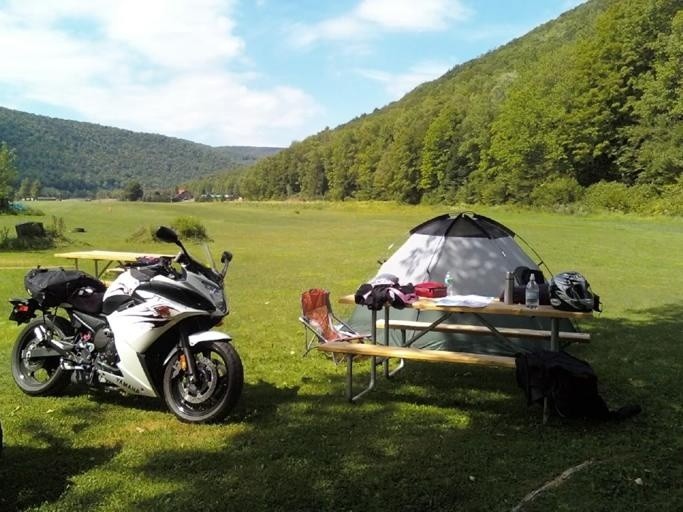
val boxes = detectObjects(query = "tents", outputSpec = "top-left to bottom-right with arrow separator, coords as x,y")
334,212 -> 580,357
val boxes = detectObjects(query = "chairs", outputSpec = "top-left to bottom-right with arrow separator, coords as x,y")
298,287 -> 373,368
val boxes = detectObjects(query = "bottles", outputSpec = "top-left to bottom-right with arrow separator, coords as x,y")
525,273 -> 540,310
443,271 -> 455,298
503,271 -> 515,306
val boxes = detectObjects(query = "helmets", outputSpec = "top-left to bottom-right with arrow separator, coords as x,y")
549,268 -> 596,314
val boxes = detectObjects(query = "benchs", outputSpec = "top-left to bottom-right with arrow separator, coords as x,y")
317,291 -> 593,425
54,251 -> 177,290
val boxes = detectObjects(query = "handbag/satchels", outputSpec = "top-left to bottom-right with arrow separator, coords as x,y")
413,282 -> 447,297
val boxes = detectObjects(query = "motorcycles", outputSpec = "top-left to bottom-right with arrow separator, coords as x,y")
9,221 -> 248,431
